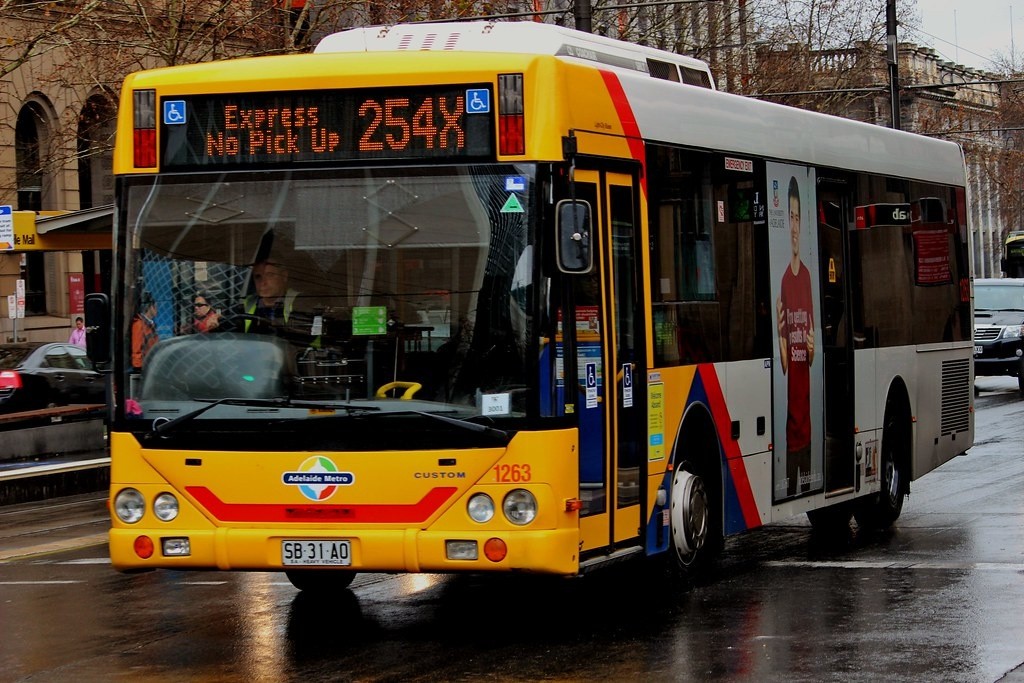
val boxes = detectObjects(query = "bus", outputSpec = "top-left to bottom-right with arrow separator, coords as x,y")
1001,230 -> 1024,278
86,20 -> 975,595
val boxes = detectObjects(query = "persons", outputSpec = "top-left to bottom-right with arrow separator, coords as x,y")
129,290 -> 160,374
177,289 -> 227,332
207,250 -> 322,348
67,317 -> 87,349
774,174 -> 815,496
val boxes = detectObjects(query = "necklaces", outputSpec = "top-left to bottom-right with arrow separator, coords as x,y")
78,330 -> 84,342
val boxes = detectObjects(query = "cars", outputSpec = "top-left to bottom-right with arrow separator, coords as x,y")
1,342 -> 108,431
973,277 -> 1024,396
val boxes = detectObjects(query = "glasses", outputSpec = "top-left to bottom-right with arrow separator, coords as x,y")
194,303 -> 209,307
